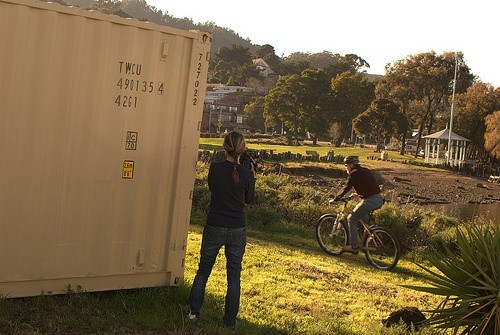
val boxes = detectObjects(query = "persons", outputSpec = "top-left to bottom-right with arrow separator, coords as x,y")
188,132 -> 257,330
328,155 -> 384,256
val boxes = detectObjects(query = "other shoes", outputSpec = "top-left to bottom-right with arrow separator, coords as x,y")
342,245 -> 358,254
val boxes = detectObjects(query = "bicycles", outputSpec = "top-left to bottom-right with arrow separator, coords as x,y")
316,195 -> 400,271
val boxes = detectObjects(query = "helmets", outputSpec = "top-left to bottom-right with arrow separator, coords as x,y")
344,155 -> 359,164
